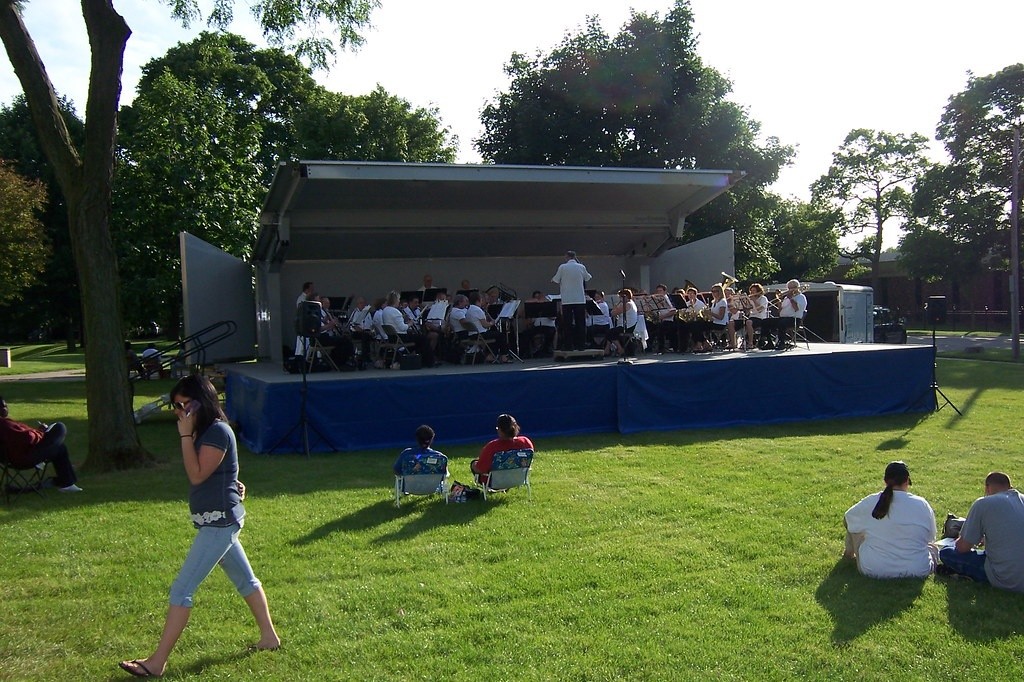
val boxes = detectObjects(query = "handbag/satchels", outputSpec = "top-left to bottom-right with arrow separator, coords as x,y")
471,488 -> 480,501
448,481 -> 472,505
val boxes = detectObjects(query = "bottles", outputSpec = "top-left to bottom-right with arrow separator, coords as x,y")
461,492 -> 467,504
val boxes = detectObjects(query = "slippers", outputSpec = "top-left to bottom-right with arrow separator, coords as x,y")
242,644 -> 283,653
117,659 -> 163,679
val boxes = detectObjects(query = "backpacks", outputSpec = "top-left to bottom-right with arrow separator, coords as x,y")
284,355 -> 310,374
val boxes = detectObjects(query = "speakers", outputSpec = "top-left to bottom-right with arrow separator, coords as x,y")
296,301 -> 323,336
928,296 -> 947,324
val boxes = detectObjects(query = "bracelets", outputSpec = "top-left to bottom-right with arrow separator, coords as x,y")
180,435 -> 193,438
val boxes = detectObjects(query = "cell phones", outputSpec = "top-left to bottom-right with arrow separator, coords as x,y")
185,400 -> 201,417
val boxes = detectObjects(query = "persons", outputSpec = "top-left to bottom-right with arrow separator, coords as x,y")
0,398 -> 83,492
470,414 -> 534,488
937,472 -> 1024,592
762,279 -> 807,350
524,289 -> 638,356
170,343 -> 185,375
842,461 -> 937,579
117,375 -> 281,676
646,283 -> 768,352
125,341 -> 140,369
294,281 -> 513,371
552,251 -> 592,351
392,425 -> 447,475
142,343 -> 161,362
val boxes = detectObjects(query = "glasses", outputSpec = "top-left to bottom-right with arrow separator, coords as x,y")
495,426 -> 500,430
171,399 -> 193,412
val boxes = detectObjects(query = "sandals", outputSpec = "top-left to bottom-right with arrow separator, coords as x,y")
500,358 -> 513,364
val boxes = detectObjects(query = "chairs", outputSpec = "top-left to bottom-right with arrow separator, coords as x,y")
297,295 -> 810,375
394,452 -> 451,506
474,448 -> 534,501
0,442 -> 50,504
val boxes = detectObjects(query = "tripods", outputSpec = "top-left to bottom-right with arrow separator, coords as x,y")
493,299 -> 525,365
644,294 -> 672,356
788,310 -> 829,350
734,297 -> 758,354
902,325 -> 963,416
267,336 -> 338,459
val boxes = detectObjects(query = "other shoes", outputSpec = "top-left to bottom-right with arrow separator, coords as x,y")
760,341 -> 774,349
712,340 -> 737,351
59,484 -> 84,493
774,341 -> 785,350
748,343 -> 754,350
658,344 -> 712,354
